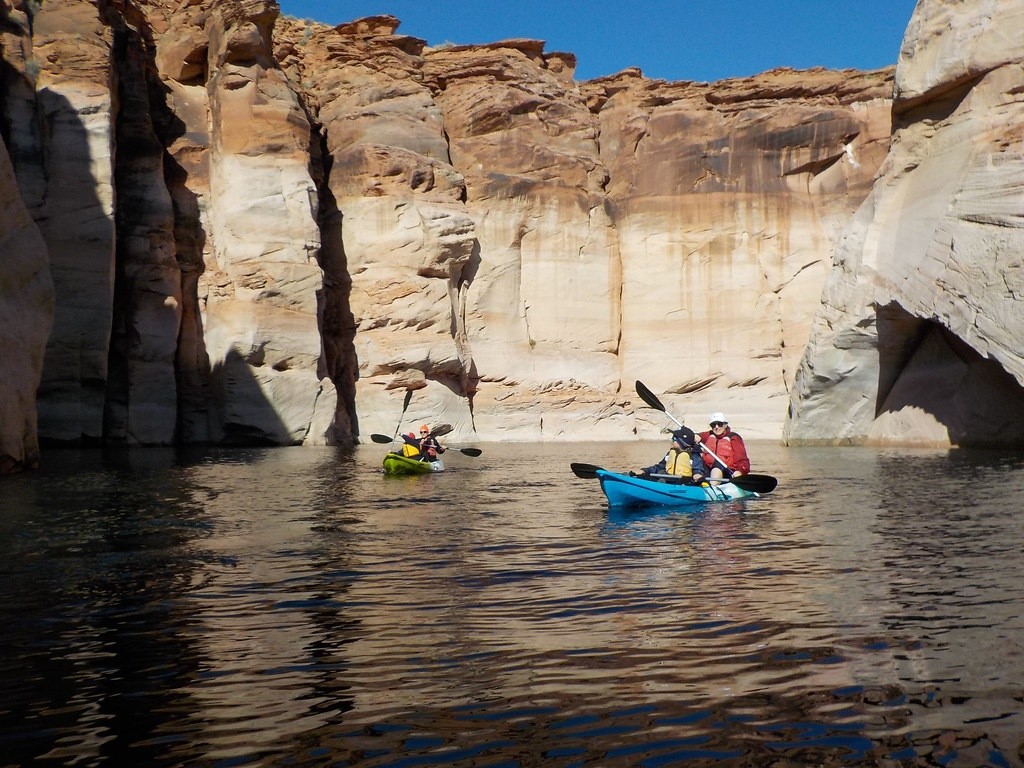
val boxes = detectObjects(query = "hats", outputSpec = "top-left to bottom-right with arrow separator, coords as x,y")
420,424 -> 429,433
709,412 -> 728,426
409,433 -> 415,440
668,427 -> 696,448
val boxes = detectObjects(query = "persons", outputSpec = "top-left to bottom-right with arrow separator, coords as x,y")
389,433 -> 420,461
693,412 -> 750,485
394,425 -> 445,462
630,427 -> 704,487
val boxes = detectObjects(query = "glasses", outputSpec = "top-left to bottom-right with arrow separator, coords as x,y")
420,431 -> 427,434
671,436 -> 677,443
710,422 -> 723,428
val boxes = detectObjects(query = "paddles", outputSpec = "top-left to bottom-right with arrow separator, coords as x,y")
370,434 -> 483,456
391,390 -> 413,447
571,462 -> 778,494
636,380 -> 760,497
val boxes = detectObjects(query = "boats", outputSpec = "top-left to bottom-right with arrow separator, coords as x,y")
596,469 -> 761,507
383,453 -> 444,474
